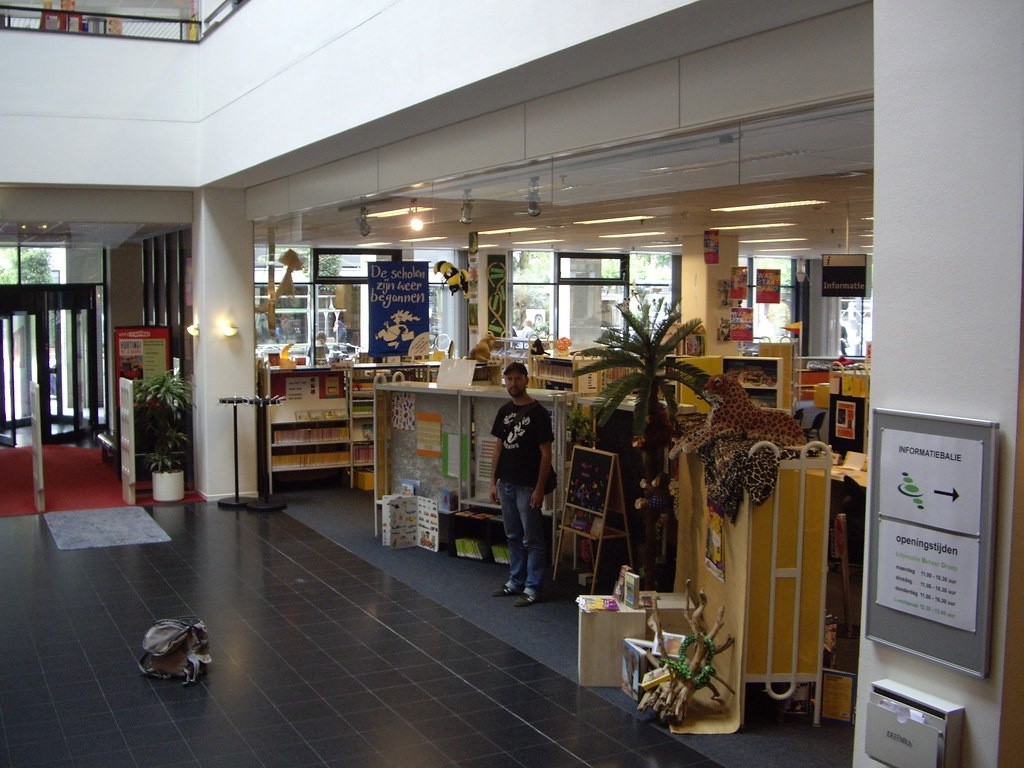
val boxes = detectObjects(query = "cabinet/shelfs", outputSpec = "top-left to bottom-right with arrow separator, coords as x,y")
494,331 -> 665,450
256,354 -> 503,496
676,336 -> 866,416
676,441 -> 834,727
828,362 -> 871,461
372,371 -> 697,567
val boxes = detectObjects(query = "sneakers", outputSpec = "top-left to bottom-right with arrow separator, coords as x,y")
512,592 -> 536,606
491,586 -> 518,597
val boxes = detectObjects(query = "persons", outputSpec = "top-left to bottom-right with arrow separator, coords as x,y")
518,321 -> 535,346
122,356 -> 143,381
489,362 -> 555,607
307,331 -> 329,365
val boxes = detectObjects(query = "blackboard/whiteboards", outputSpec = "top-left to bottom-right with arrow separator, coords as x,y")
565,446 -> 613,516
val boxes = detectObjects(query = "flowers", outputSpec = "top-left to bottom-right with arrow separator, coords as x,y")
556,337 -> 572,350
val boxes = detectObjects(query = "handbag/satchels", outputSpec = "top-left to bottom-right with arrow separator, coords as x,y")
140,615 -> 210,684
532,460 -> 558,495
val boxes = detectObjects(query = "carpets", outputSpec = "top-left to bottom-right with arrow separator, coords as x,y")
0,443 -> 207,517
42,506 -> 171,549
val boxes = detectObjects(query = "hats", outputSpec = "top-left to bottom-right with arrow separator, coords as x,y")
504,362 -> 528,375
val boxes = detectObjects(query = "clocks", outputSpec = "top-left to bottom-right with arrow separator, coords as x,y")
434,334 -> 451,351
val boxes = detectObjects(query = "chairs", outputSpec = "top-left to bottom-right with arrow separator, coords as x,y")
803,412 -> 826,443
793,408 -> 804,426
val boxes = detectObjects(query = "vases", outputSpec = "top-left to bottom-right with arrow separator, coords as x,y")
558,348 -> 569,356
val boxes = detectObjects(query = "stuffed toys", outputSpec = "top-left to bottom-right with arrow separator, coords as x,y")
632,412 -> 672,469
634,472 -> 673,510
470,337 -> 495,361
667,374 -> 804,460
434,261 -> 470,299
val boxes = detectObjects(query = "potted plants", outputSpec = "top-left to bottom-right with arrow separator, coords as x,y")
133,369 -> 198,501
566,404 -> 599,461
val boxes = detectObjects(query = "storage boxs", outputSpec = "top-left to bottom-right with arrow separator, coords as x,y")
358,472 -> 373,490
489,512 -> 562,567
577,595 -> 646,686
449,506 -> 501,562
645,593 -> 696,641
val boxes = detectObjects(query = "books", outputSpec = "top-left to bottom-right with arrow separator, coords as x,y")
538,364 -> 572,391
614,565 -> 639,609
272,425 -> 374,469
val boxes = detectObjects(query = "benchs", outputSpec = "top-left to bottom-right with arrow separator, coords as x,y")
98,434 -> 118,471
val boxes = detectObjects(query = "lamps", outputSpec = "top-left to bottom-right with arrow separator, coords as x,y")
187,325 -> 200,335
458,188 -> 473,224
526,175 -> 541,217
224,327 -> 238,337
355,206 -> 372,236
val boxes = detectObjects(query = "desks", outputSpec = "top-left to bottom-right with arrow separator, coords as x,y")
794,464 -> 867,487
